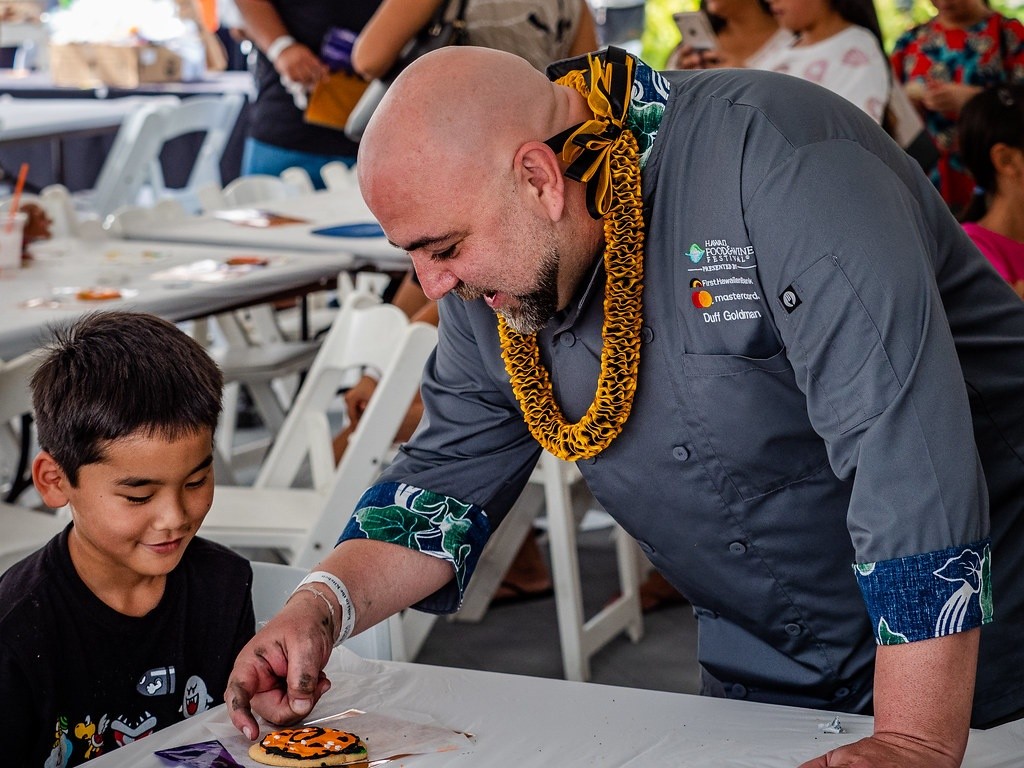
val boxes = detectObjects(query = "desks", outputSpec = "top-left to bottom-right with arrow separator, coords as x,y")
74,658 -> 1024,768
0,97 -> 173,183
1,69 -> 253,191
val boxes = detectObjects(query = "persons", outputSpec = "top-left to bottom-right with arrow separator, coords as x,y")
0,309 -> 255,768
228,44 -> 1024,768
178,0 -> 1024,291
329,269 -> 439,469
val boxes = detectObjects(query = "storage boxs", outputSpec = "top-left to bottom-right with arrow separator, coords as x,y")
49,42 -> 182,90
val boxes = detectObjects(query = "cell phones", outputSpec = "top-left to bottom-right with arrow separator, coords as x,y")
671,11 -> 722,66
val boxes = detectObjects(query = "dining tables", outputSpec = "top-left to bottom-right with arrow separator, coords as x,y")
0,241 -> 349,503
130,191 -> 413,262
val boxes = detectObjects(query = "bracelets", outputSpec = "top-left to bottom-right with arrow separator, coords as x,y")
363,367 -> 380,382
283,571 -> 355,647
265,36 -> 295,60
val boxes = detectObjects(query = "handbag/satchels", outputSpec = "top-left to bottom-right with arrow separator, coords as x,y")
344,75 -> 390,143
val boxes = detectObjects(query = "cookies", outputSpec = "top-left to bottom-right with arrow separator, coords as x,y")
248,727 -> 368,768
226,257 -> 268,265
75,286 -> 121,299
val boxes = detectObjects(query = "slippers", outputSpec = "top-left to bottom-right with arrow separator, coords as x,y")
600,587 -> 686,614
488,581 -> 553,610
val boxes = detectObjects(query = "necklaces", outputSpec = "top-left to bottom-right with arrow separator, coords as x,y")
498,69 -> 643,462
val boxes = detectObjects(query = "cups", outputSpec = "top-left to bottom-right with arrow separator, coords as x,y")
0,211 -> 28,269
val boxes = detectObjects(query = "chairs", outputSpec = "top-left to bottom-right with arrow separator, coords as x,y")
447,450 -> 643,684
51,290 -> 440,569
104,160 -> 358,233
89,90 -> 287,433
